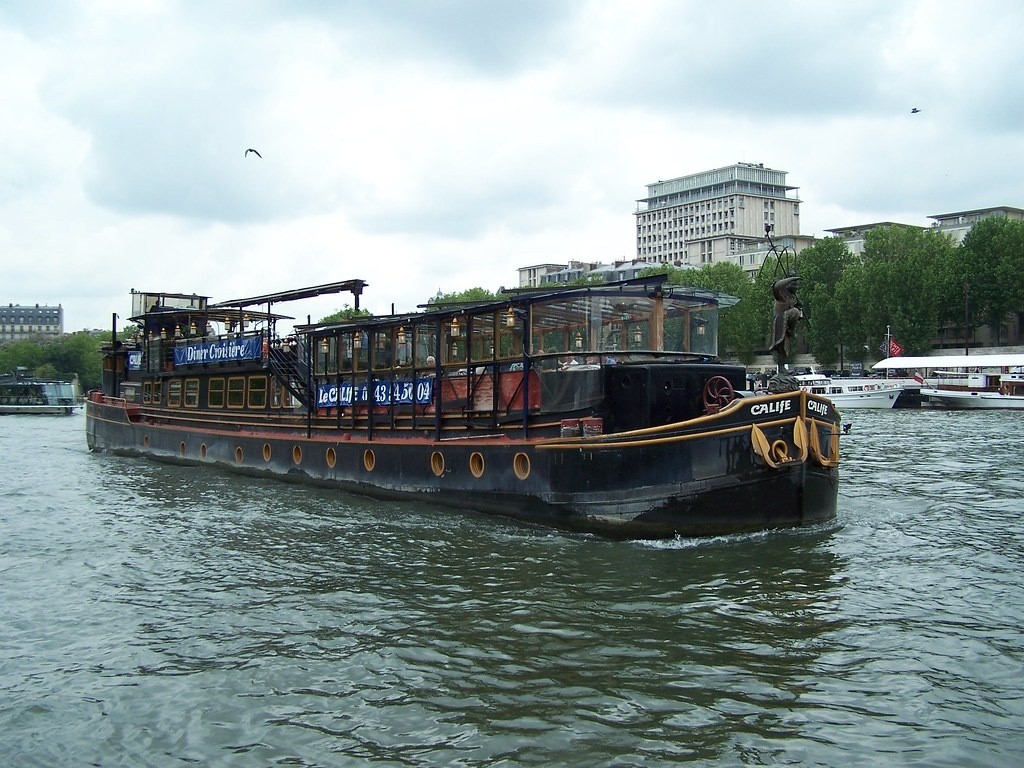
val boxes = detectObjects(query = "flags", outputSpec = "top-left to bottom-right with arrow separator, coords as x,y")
891,336 -> 904,357
914,372 -> 924,384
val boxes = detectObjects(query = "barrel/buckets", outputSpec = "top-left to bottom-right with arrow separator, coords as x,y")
561,419 -> 580,437
582,418 -> 602,436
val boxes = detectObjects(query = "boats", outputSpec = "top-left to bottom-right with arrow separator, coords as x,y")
81,221 -> 857,540
0,366 -> 85,416
919,369 -> 1024,410
794,324 -> 904,410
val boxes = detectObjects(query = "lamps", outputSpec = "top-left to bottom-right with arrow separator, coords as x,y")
148,331 -> 154,341
489,343 -> 494,356
243,313 -> 250,327
160,328 -> 166,339
696,322 -> 706,335
224,317 -> 231,330
320,338 -> 330,354
575,332 -> 583,348
206,322 -> 212,333
397,326 -> 406,344
452,342 -> 458,356
450,317 -> 460,337
506,307 -> 516,328
633,326 -> 643,343
174,325 -> 181,336
354,332 -> 362,349
190,323 -> 196,335
282,338 -> 290,353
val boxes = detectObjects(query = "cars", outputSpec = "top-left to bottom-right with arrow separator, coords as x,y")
746,364 -> 910,381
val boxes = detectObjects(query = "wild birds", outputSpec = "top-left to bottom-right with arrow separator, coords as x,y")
245,149 -> 262,158
911,107 -> 920,113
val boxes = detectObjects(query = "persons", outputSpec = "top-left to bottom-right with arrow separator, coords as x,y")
759,244 -> 813,373
394,347 -> 617,379
